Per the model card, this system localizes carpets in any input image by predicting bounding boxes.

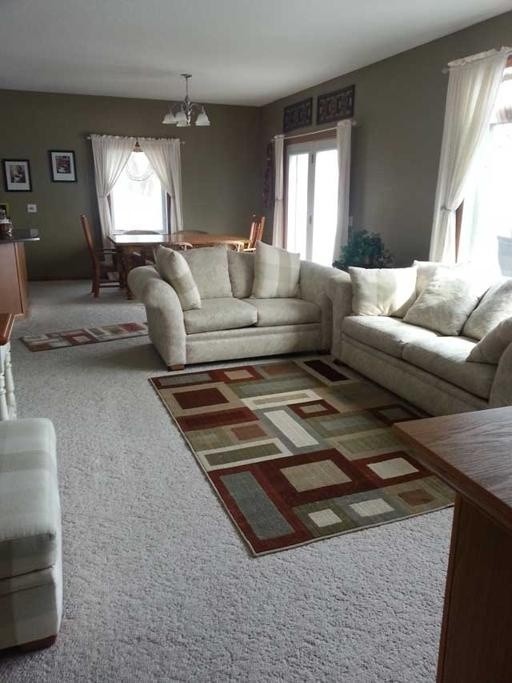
[148,351,458,557]
[19,319,149,352]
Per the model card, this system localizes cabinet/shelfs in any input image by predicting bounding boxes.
[391,405,512,682]
[1,240,30,318]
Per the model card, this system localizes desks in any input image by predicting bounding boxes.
[1,311,17,420]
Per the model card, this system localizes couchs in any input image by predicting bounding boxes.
[330,258,512,416]
[127,238,350,371]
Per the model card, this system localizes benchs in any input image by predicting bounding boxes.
[0,418,65,655]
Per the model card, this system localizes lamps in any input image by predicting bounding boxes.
[160,72,212,129]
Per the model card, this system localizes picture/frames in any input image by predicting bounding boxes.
[0,201,9,216]
[3,156,32,194]
[48,148,79,185]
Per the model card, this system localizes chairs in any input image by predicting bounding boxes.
[201,239,241,250]
[79,213,128,298]
[171,229,206,234]
[154,240,194,261]
[122,229,160,260]
[244,213,267,251]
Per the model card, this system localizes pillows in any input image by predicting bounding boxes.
[463,278,512,342]
[465,315,512,364]
[402,265,490,337]
[347,265,418,318]
[153,242,201,312]
[250,241,302,300]
